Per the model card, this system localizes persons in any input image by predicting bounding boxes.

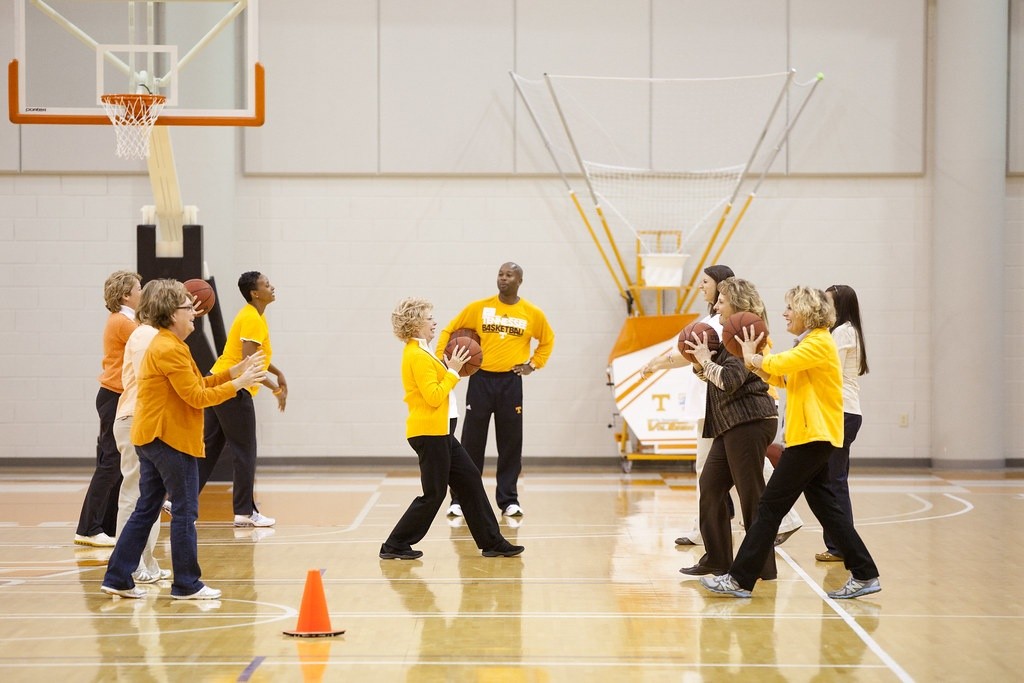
[675,265,804,547]
[99,281,267,600]
[815,285,869,561]
[378,298,525,560]
[163,271,288,527]
[697,286,881,604]
[74,271,143,547]
[113,278,204,583]
[678,277,777,581]
[434,262,556,514]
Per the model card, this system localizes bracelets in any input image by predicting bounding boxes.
[273,388,283,395]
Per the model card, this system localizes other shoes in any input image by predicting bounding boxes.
[674,537,695,545]
[814,551,844,562]
[773,526,803,547]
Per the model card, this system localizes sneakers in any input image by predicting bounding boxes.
[170,585,222,600]
[446,504,463,516]
[700,573,752,598]
[827,575,882,600]
[131,568,160,584]
[481,538,525,557]
[100,584,149,598]
[502,503,525,516]
[379,542,423,559]
[152,567,172,579]
[162,500,197,525]
[73,532,116,547]
[233,508,276,527]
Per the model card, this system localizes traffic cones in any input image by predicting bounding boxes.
[280,568,345,641]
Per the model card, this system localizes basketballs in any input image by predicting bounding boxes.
[678,322,720,363]
[722,311,768,357]
[443,337,483,377]
[448,327,481,346]
[182,279,216,319]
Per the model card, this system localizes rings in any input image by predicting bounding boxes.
[254,363,257,367]
[521,368,523,371]
[255,378,257,382]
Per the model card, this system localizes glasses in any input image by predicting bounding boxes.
[174,304,194,312]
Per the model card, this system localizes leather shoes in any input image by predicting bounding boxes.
[679,563,728,576]
